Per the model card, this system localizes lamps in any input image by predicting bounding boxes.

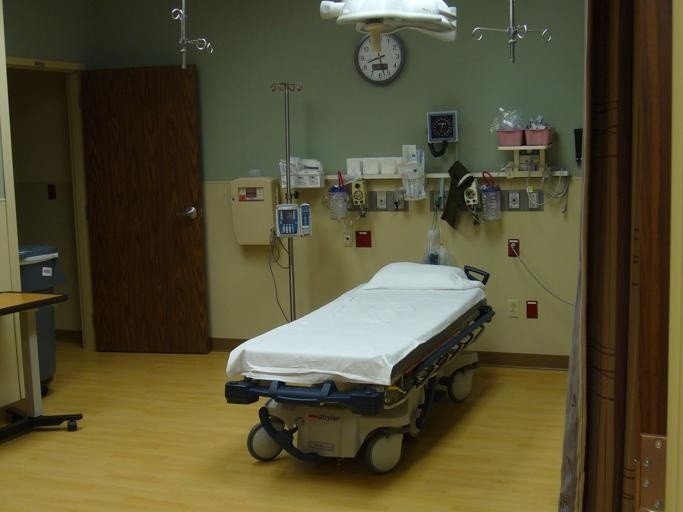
[319,0,457,42]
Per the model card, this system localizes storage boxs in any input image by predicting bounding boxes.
[496,130,525,146]
[525,128,553,145]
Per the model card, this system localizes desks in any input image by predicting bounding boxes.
[0,290,82,444]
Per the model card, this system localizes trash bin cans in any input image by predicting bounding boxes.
[17,244,59,396]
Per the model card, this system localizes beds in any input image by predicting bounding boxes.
[225,265,496,475]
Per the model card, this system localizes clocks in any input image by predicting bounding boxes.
[427,110,458,143]
[353,31,406,84]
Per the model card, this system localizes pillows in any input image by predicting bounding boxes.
[363,261,487,290]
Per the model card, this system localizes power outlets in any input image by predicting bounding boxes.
[507,239,519,257]
[433,195,443,208]
